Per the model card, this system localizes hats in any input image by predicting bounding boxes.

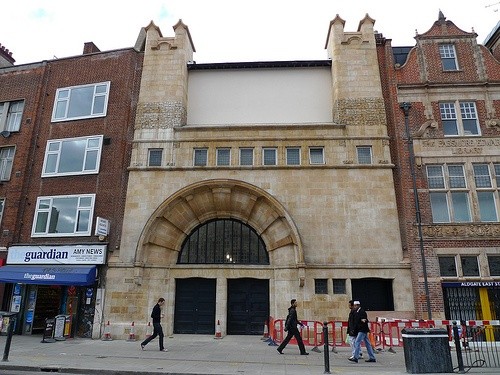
[354,301,360,305]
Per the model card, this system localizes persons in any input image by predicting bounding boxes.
[277,300,309,354]
[141,298,168,352]
[348,300,363,359]
[348,301,377,363]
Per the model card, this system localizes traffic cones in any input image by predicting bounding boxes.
[144,321,153,339]
[261,319,269,340]
[101,321,113,341]
[126,322,138,342]
[214,319,223,340]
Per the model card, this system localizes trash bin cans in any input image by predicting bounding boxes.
[0,311,17,336]
[401,327,454,373]
[53,314,72,341]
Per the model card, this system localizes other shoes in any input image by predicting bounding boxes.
[277,348,284,354]
[350,354,364,358]
[300,352,309,355]
[141,343,146,350]
[160,348,168,352]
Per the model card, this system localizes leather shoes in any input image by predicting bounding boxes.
[349,357,358,362]
[366,358,376,362]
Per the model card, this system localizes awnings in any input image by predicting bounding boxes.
[0,265,96,287]
